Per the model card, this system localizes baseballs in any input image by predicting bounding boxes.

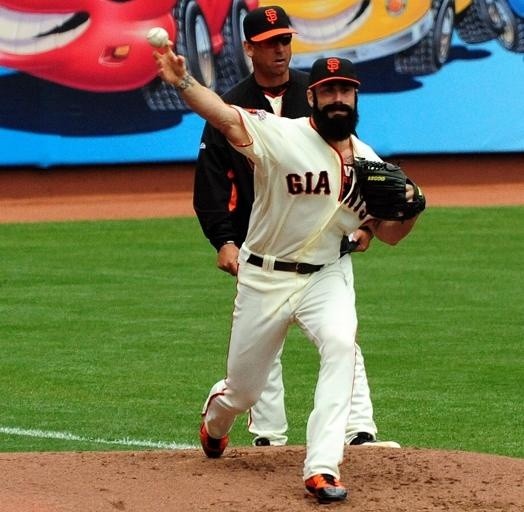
[148,27,168,49]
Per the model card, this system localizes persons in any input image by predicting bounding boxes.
[192,5,403,449]
[151,44,426,502]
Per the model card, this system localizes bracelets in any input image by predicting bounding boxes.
[223,240,235,244]
[176,73,192,92]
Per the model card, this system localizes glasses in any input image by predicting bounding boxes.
[256,35,291,48]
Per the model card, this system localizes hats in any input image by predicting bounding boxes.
[243,6,298,43]
[307,57,360,90]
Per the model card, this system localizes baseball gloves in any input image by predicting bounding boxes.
[351,158,425,222]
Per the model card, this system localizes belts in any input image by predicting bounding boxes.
[247,235,350,273]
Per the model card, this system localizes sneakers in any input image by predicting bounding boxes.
[304,474,347,498]
[348,432,401,448]
[200,420,229,459]
